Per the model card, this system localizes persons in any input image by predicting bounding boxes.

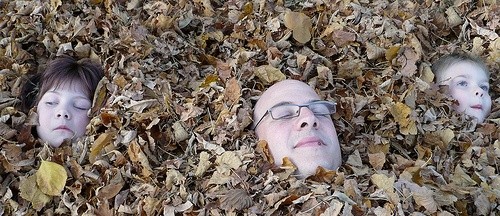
[33,53,105,149]
[251,79,342,182]
[430,54,493,126]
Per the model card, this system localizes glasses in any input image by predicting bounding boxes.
[252,101,335,136]
